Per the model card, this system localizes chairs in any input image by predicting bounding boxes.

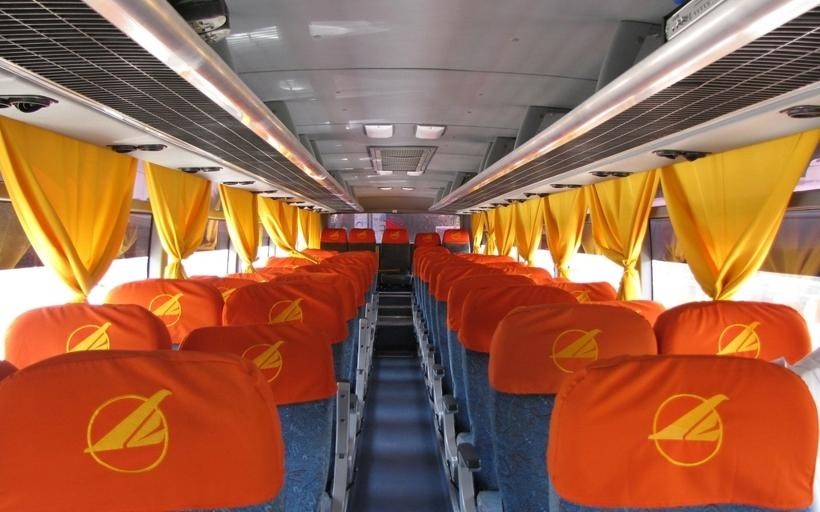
[379,229,412,288]
[2,247,380,511]
[414,231,441,245]
[409,245,819,512]
[319,228,348,252]
[346,229,377,250]
[443,229,472,253]
